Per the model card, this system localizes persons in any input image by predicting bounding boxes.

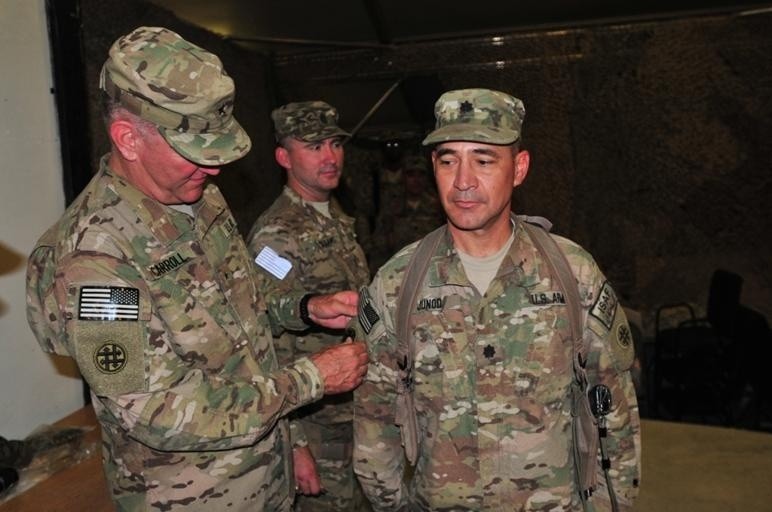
[349,87,642,511]
[25,27,369,511]
[372,155,446,277]
[245,101,376,511]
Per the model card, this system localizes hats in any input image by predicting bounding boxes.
[271,101,354,143]
[98,26,253,168]
[422,89,527,147]
